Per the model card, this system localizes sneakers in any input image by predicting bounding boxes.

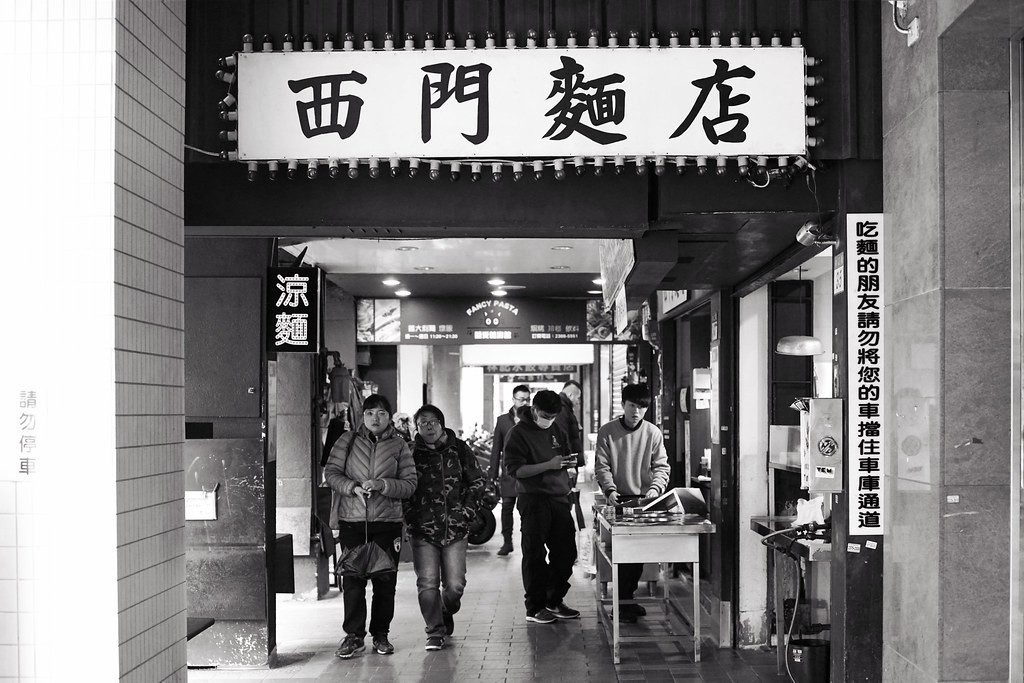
[334,632,365,658]
[525,606,557,624]
[372,634,394,655]
[544,601,580,618]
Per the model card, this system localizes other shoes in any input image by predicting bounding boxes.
[611,603,646,623]
[425,635,443,650]
[498,540,514,555]
[442,608,454,636]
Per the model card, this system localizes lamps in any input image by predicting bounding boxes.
[774,265,827,356]
[796,218,843,250]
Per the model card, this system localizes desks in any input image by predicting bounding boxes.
[592,504,716,665]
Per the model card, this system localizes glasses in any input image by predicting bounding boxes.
[363,409,388,418]
[417,418,441,428]
[514,396,531,402]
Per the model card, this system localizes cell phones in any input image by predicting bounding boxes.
[568,453,579,462]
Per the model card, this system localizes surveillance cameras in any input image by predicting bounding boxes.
[796,221,821,246]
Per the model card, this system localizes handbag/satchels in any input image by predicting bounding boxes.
[329,489,342,529]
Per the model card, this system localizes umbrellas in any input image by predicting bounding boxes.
[333,494,399,575]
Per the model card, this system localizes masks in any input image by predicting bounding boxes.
[533,409,556,430]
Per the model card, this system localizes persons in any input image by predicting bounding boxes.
[595,384,671,624]
[555,380,585,466]
[325,394,417,658]
[488,385,530,557]
[400,404,486,650]
[503,390,579,623]
